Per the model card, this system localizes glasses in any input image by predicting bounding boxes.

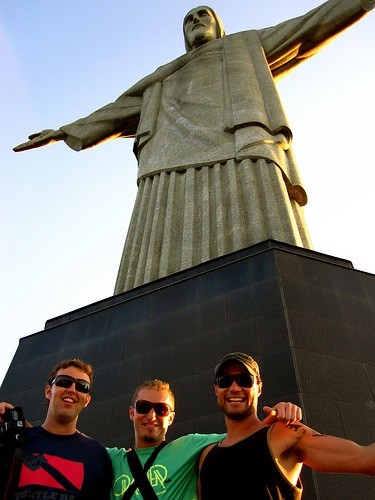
[51,375,92,393]
[215,374,259,388]
[134,399,173,416]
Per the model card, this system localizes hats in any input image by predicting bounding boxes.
[214,352,260,380]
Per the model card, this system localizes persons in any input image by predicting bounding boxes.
[195,352,375,500]
[0,377,302,500]
[11,0,375,296]
[0,357,115,500]
